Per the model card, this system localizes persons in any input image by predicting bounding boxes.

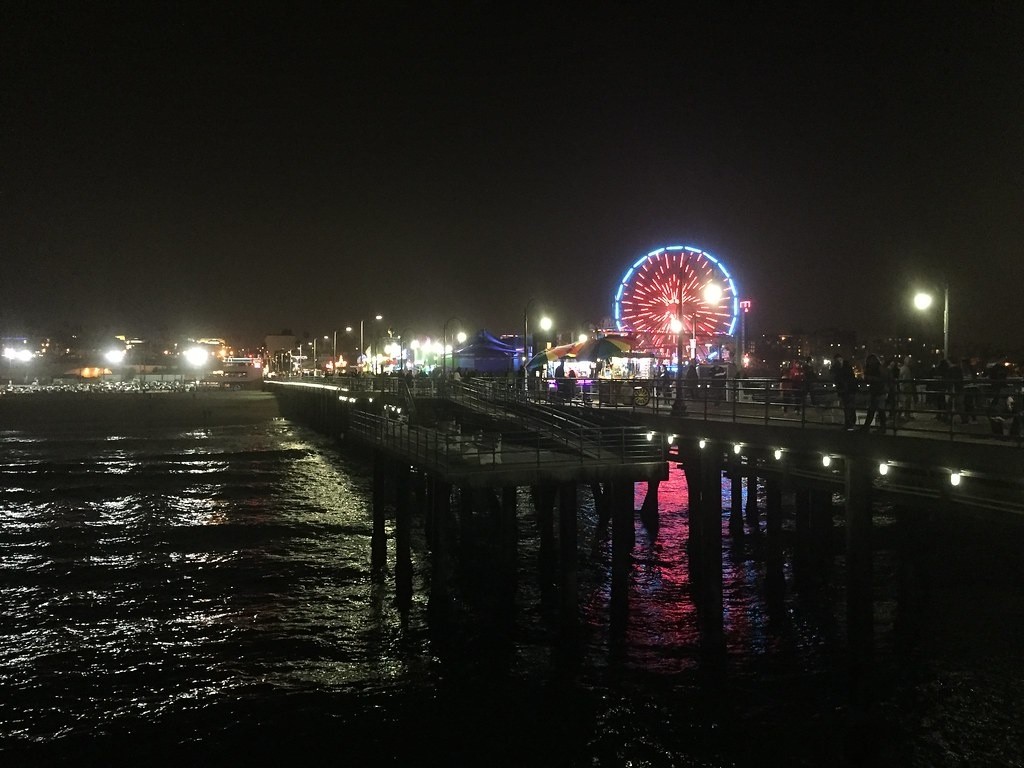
[311,345,1024,441]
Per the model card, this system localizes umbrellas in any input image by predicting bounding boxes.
[523,333,641,380]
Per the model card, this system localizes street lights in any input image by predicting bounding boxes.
[401,328,420,372]
[314,335,328,371]
[443,316,468,385]
[333,325,352,376]
[290,341,312,377]
[913,280,948,364]
[361,315,383,378]
[524,297,552,369]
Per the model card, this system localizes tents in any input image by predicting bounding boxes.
[446,328,520,375]
